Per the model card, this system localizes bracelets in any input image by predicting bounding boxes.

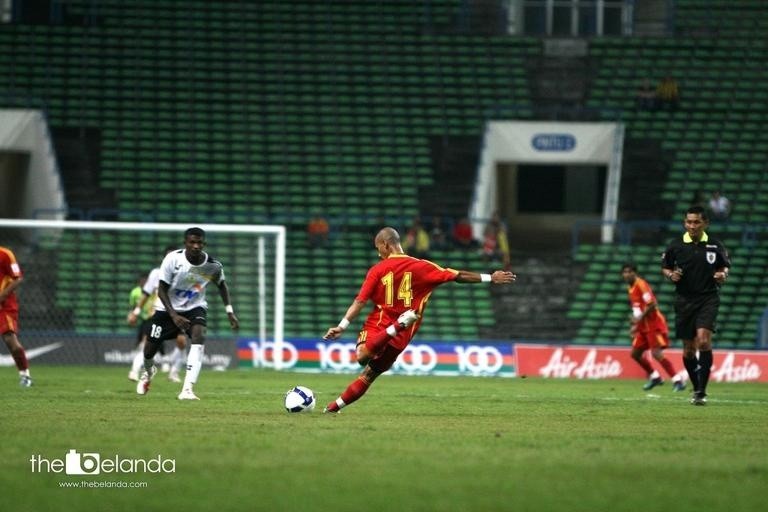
[667,271,672,279]
[338,318,350,330]
[480,273,491,282]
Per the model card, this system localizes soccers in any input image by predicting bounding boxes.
[284,386,315,413]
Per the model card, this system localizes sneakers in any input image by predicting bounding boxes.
[323,404,342,415]
[671,376,688,395]
[136,366,159,395]
[127,368,140,382]
[643,376,665,391]
[397,307,419,333]
[176,388,201,403]
[690,392,708,407]
[168,372,182,385]
[19,373,33,391]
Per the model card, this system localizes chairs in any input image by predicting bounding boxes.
[0,0,767,351]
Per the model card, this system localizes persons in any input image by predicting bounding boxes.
[660,207,732,405]
[621,263,687,392]
[323,227,516,413]
[365,211,503,263]
[705,190,734,224]
[128,227,239,400]
[0,247,32,387]
